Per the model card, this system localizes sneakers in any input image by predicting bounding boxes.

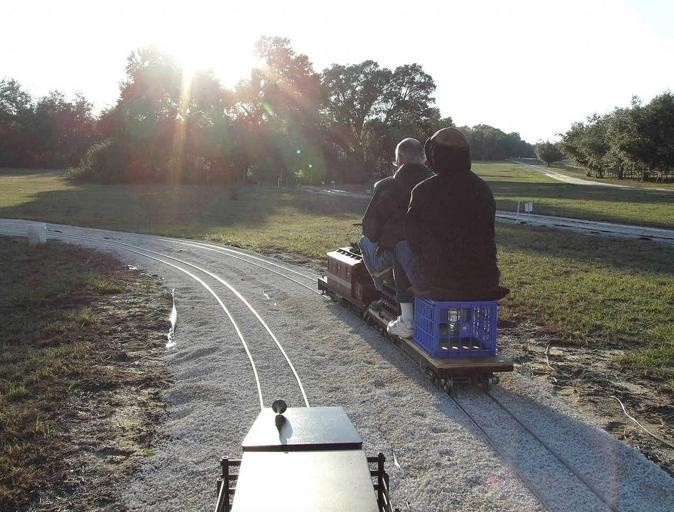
[387,315,416,338]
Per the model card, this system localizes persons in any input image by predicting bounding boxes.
[356,126,511,339]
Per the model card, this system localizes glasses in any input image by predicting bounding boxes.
[393,158,399,167]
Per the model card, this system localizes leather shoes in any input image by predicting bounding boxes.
[371,298,384,311]
